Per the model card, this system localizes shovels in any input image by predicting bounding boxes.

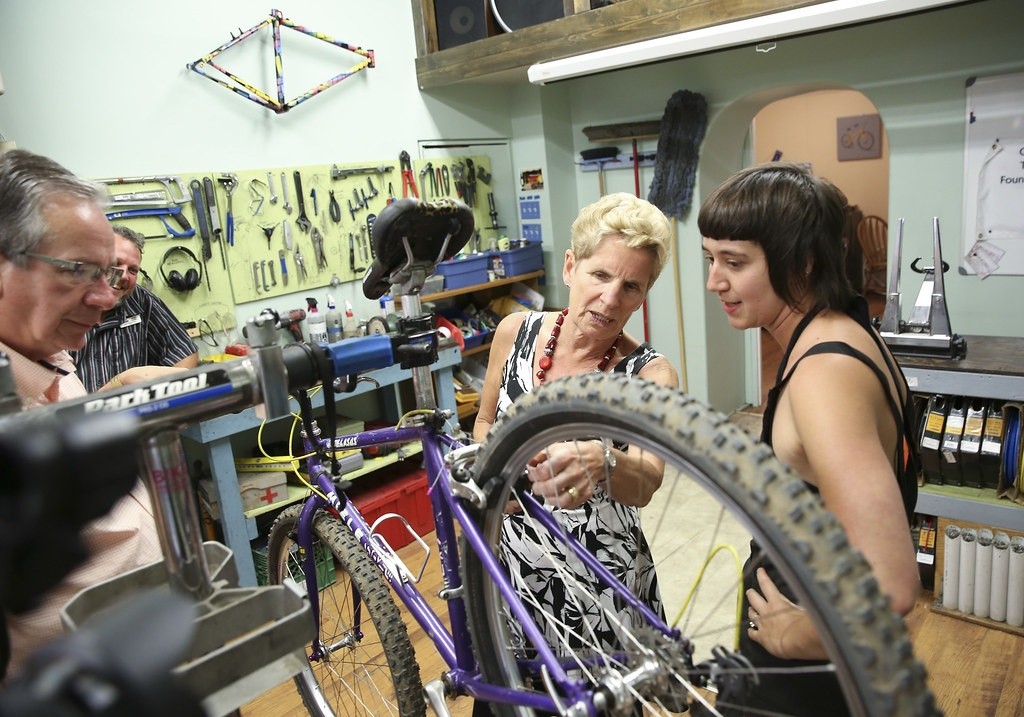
[327,190,342,222]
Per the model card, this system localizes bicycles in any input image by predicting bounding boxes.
[1,197,941,717]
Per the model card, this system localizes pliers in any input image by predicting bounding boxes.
[399,149,419,200]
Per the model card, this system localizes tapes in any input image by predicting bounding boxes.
[437,326,451,339]
[449,318,480,339]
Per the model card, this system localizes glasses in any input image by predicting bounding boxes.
[21,252,123,288]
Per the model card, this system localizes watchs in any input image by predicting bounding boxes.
[587,439,617,486]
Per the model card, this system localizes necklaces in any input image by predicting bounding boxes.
[536,308,622,387]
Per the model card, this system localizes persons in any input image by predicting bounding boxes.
[698,165,918,716]
[0,149,199,676]
[471,194,680,717]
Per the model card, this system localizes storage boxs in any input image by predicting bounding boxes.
[432,252,489,290]
[252,537,336,600]
[338,465,438,551]
[485,240,544,277]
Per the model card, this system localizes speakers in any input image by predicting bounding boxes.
[433,0,503,52]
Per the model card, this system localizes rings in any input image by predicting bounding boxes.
[566,486,580,508]
[749,614,757,627]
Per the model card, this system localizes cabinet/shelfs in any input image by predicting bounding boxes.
[389,270,546,422]
[176,343,462,590]
[868,336,1024,633]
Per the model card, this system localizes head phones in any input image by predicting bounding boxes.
[160,246,202,292]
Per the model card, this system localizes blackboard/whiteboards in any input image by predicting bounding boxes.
[958,71,1024,279]
